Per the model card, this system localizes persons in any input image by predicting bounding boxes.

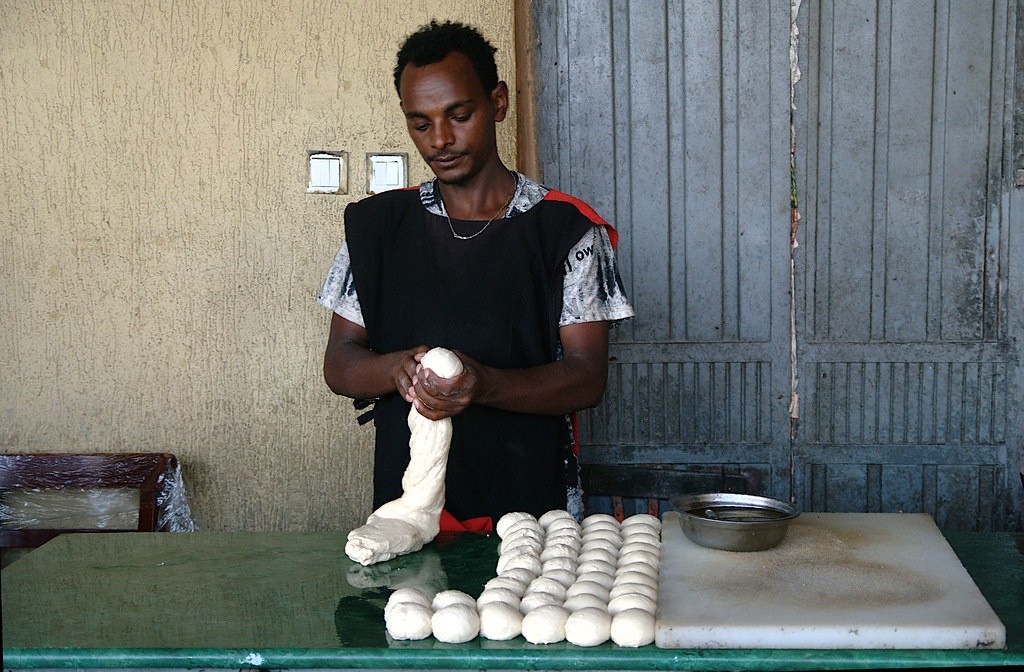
[317,19,635,534]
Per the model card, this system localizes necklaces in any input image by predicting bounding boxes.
[448,173,516,239]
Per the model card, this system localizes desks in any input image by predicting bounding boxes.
[2,531,1024,669]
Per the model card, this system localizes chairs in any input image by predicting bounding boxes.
[0,451,179,569]
[578,462,749,523]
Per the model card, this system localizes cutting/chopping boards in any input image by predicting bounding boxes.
[655,510,1005,650]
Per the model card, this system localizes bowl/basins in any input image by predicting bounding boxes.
[667,491,801,552]
